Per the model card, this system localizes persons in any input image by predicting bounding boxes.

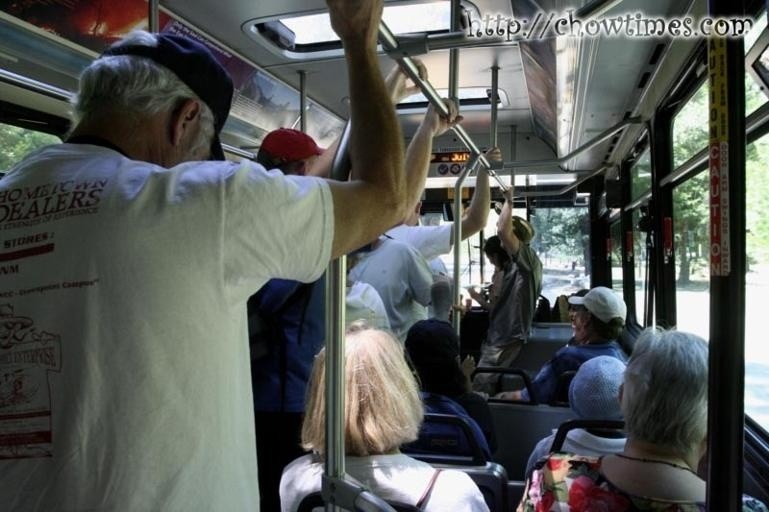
[341,97,630,406]
[513,324,766,510]
[2,2,408,511]
[397,319,495,448]
[245,56,470,509]
[524,355,629,481]
[279,320,491,512]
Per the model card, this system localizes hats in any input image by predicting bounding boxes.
[567,285,629,327]
[261,128,323,159]
[98,32,235,163]
[511,215,535,242]
[570,354,627,420]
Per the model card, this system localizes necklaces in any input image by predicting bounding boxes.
[614,452,699,474]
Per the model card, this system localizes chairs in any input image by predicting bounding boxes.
[295,365,769,512]
